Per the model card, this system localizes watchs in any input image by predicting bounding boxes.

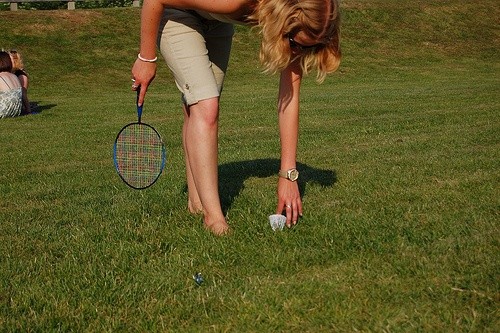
[278,168,299,182]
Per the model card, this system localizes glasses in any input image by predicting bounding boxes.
[289,32,321,50]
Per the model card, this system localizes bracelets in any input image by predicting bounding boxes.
[137,53,158,62]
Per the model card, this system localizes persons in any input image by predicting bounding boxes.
[0,50,30,118]
[130,0,342,238]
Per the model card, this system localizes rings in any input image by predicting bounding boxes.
[284,205,292,208]
[132,85,137,88]
[131,79,135,81]
